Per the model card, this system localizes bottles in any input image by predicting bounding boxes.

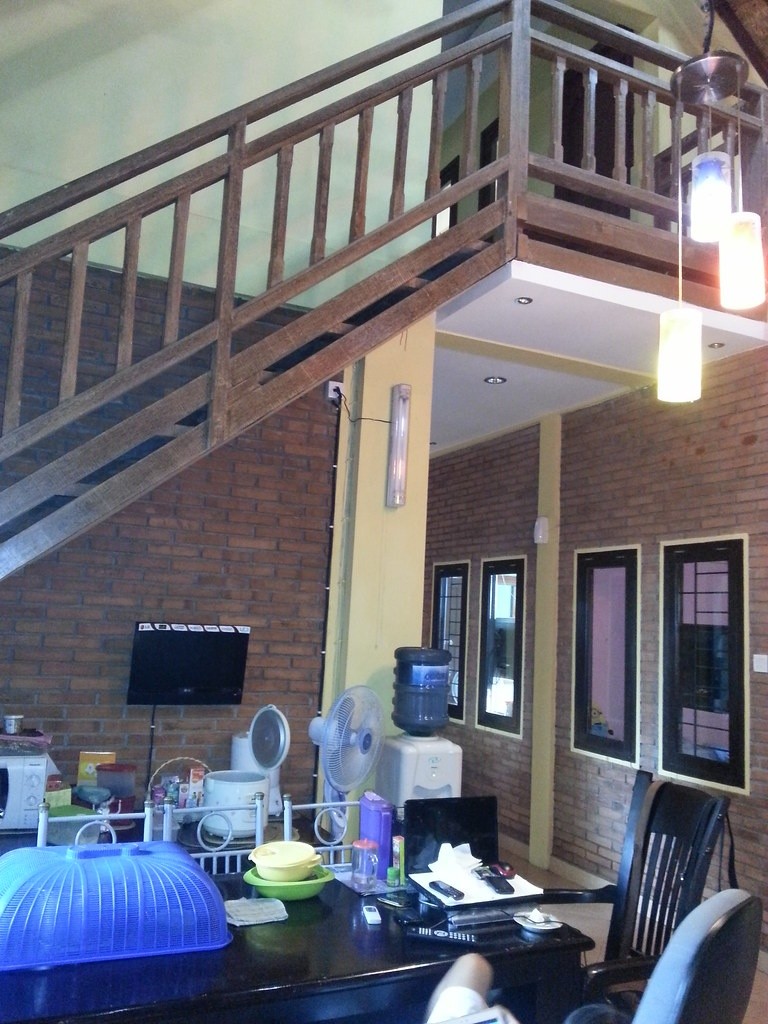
[392,647,452,736]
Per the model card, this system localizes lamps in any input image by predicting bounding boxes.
[386,383,412,508]
[656,0,766,403]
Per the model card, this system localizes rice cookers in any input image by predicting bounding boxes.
[203,704,291,840]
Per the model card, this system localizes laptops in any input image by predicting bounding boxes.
[402,795,545,909]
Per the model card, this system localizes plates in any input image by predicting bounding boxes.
[514,912,563,932]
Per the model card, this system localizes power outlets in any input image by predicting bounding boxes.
[325,380,343,401]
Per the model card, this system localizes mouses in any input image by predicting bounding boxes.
[489,862,516,879]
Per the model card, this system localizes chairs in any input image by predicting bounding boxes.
[564,888,763,1024]
[163,791,265,874]
[534,769,730,1024]
[283,789,374,864]
[37,801,155,847]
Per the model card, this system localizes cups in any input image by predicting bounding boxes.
[353,840,379,893]
[3,714,23,736]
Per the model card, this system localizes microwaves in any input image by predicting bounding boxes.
[0,747,47,829]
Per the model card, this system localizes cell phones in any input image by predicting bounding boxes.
[394,907,423,926]
[429,881,466,901]
[486,876,515,894]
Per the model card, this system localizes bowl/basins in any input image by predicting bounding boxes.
[243,865,335,901]
[48,806,101,846]
[248,841,321,881]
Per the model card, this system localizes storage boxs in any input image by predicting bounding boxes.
[45,780,72,807]
[182,765,205,788]
[46,805,103,846]
[178,782,190,808]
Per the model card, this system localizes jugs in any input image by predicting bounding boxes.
[359,791,392,880]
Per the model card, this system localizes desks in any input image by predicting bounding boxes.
[0,865,596,1024]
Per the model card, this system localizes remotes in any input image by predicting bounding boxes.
[363,906,382,925]
[406,926,479,946]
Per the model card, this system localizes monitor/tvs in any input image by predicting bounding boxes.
[127,622,250,706]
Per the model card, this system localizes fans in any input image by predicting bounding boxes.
[308,684,384,864]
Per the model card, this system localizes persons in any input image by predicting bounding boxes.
[425,954,631,1024]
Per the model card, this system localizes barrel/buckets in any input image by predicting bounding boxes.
[96,764,136,831]
